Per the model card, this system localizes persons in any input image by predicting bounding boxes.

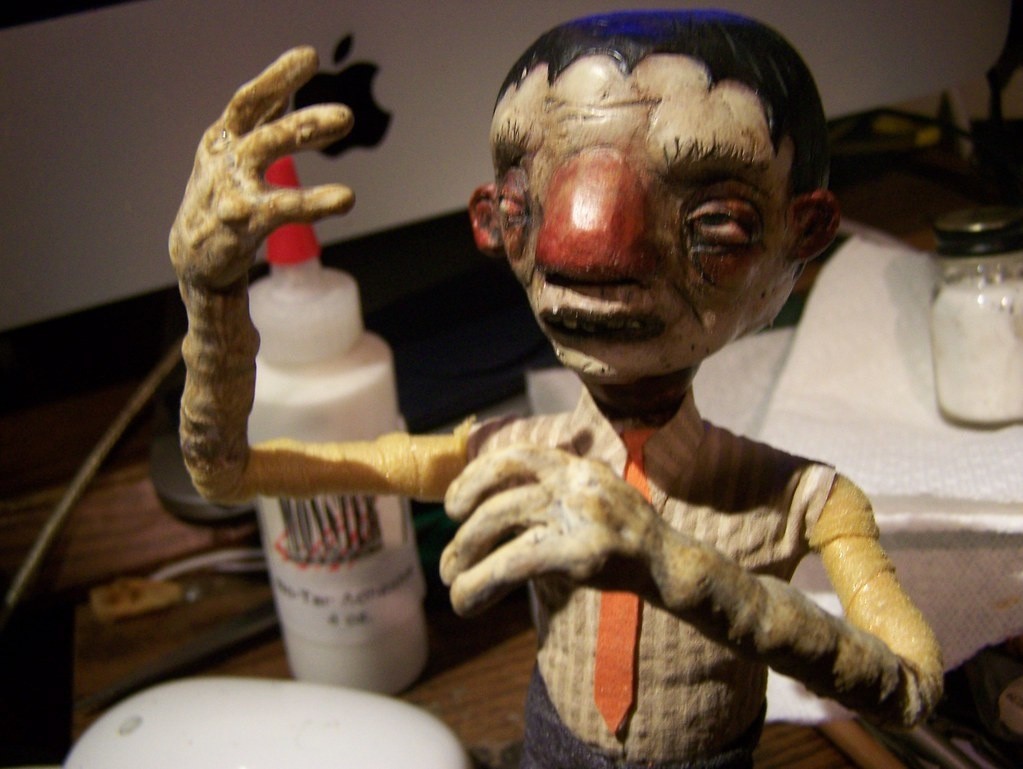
[166,9,945,769]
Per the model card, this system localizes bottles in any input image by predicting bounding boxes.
[929,208,1023,427]
[240,155,427,693]
